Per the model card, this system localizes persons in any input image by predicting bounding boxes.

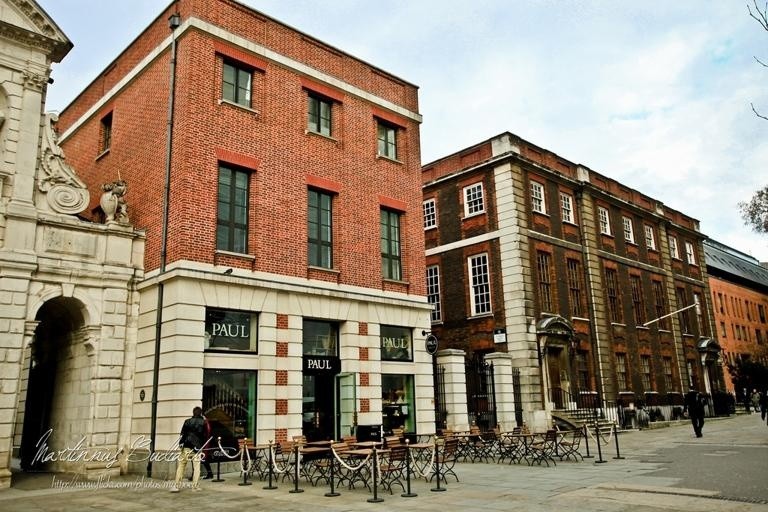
[170,407,206,493]
[741,388,751,412]
[202,415,213,479]
[759,391,768,420]
[683,386,704,437]
[750,388,760,413]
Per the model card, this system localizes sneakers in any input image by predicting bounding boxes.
[191,488,202,492]
[171,487,179,492]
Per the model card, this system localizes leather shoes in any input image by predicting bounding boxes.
[203,474,213,479]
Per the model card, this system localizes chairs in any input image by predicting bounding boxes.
[237,424,585,495]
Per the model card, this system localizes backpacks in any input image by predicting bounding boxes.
[696,392,707,406]
